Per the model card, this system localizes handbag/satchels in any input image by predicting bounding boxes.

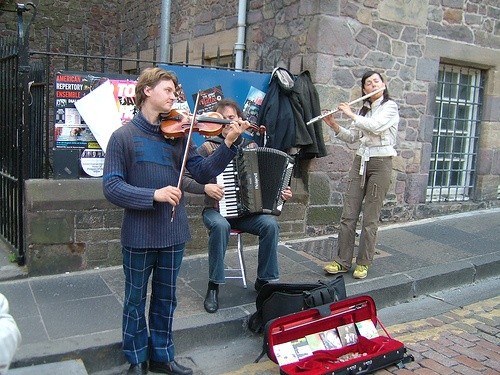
[247,275,347,335]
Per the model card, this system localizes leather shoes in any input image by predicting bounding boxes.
[149,359,193,375]
[203,289,220,313]
[254,278,269,291]
[126,360,148,375]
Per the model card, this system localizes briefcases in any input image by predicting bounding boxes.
[256,294,407,375]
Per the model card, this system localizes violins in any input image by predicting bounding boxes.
[158,108,267,140]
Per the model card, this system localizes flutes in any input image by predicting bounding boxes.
[308,87,386,126]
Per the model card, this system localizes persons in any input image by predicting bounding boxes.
[182,99,292,313]
[103,67,250,375]
[321,71,399,279]
[0,292,21,375]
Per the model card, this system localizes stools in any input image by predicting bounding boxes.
[208,228,247,289]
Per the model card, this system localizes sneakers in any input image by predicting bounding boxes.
[352,265,368,279]
[324,260,348,274]
